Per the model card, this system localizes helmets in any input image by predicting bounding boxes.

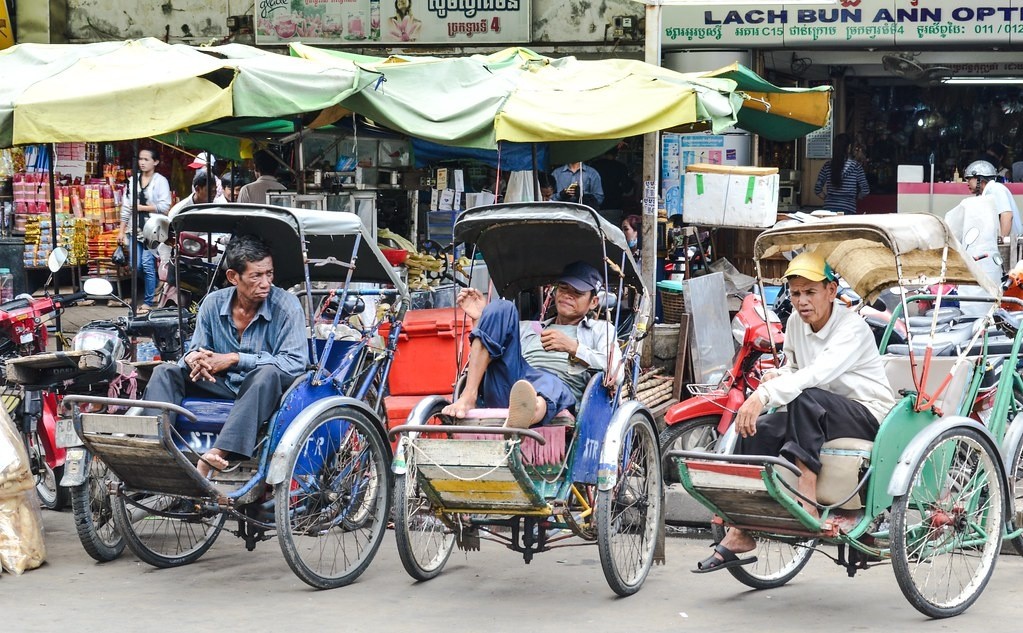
[964,160,998,176]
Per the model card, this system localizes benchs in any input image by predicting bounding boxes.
[454,370,614,462]
[773,354,967,513]
[174,342,363,426]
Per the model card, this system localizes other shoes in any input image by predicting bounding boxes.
[504,380,537,445]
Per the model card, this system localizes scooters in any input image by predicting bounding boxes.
[653,285,790,488]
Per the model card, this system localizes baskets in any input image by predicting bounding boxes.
[659,289,686,324]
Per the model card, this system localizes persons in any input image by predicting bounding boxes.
[117,146,290,314]
[386,0,422,43]
[551,161,604,208]
[814,133,871,217]
[143,236,309,481]
[534,173,557,201]
[441,260,625,443]
[621,215,643,284]
[695,253,898,573]
[964,160,1023,238]
[479,163,509,204]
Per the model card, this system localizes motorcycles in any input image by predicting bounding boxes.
[0,245,206,561]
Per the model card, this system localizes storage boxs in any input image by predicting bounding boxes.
[382,396,453,454]
[683,158,780,228]
[382,308,479,391]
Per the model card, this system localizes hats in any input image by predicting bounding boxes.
[557,262,604,295]
[187,152,216,169]
[780,252,834,282]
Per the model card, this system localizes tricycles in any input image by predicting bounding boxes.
[54,201,416,594]
[675,211,1023,619]
[379,204,665,597]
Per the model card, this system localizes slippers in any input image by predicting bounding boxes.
[690,544,758,574]
[137,307,151,315]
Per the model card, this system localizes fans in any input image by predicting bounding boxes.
[914,63,954,88]
[881,46,923,79]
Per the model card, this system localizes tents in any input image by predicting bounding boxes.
[0,37,835,156]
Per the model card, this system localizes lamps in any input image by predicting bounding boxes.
[941,76,1023,84]
